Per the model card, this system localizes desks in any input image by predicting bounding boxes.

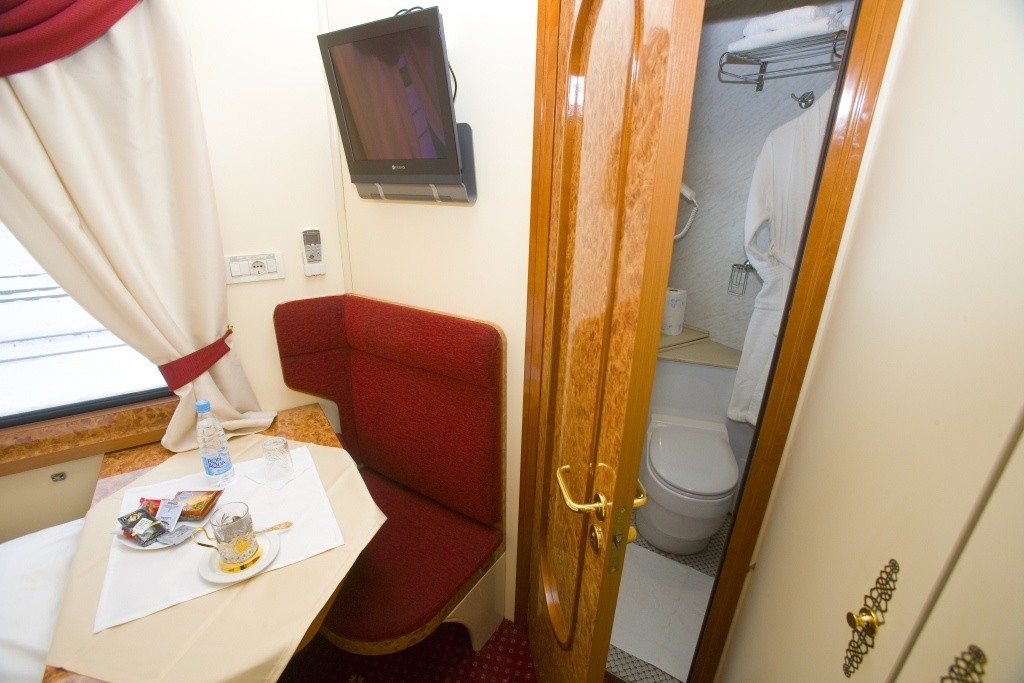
[654,320,742,367]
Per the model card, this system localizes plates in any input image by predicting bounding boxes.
[196,527,280,583]
[114,495,218,549]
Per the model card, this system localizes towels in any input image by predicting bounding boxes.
[742,6,834,37]
[727,15,844,63]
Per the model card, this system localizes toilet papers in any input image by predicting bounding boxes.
[660,287,688,336]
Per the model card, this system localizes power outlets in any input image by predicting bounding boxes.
[248,253,268,280]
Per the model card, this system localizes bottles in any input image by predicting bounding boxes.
[196,399,235,494]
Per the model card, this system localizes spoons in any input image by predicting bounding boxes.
[252,521,293,537]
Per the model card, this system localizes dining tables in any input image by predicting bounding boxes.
[43,404,384,683]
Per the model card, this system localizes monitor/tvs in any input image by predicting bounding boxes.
[317,6,464,185]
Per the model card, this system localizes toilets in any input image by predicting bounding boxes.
[638,413,741,556]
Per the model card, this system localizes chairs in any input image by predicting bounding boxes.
[274,291,508,657]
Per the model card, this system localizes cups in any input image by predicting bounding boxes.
[191,502,258,564]
[261,436,296,484]
